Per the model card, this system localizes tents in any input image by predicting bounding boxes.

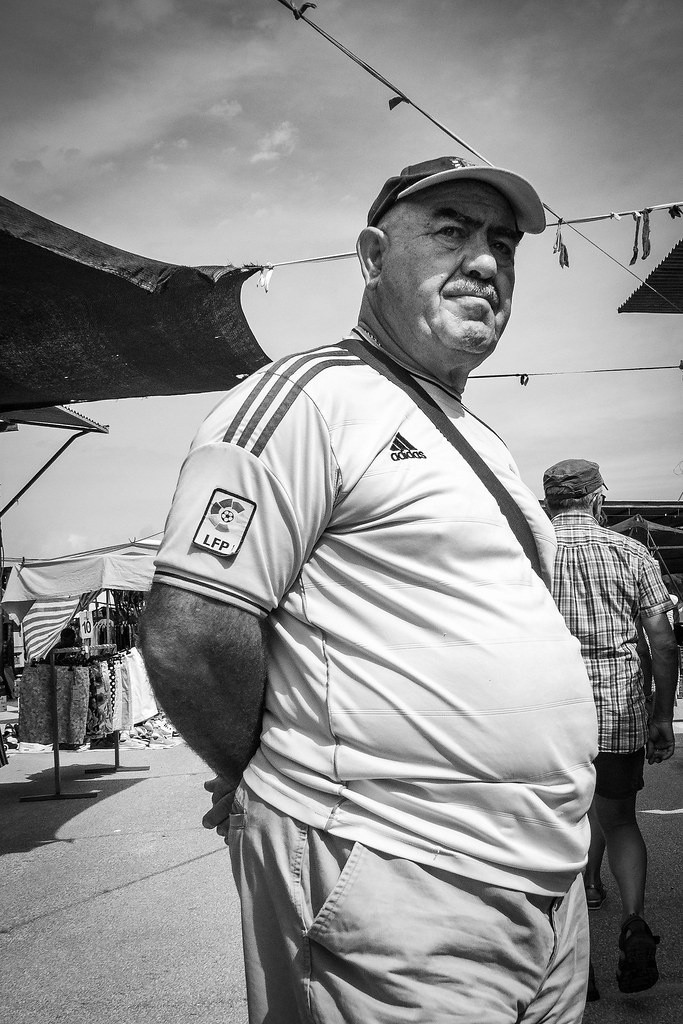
[1,538,165,655]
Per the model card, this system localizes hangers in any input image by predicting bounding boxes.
[30,645,131,668]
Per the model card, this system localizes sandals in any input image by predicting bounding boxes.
[587,959,600,1002]
[616,916,658,992]
[585,883,607,909]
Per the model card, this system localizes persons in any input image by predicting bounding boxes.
[44,628,79,666]
[141,154,604,1024]
[583,791,608,911]
[528,460,681,1001]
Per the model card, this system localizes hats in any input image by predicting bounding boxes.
[543,459,608,499]
[368,156,547,243]
